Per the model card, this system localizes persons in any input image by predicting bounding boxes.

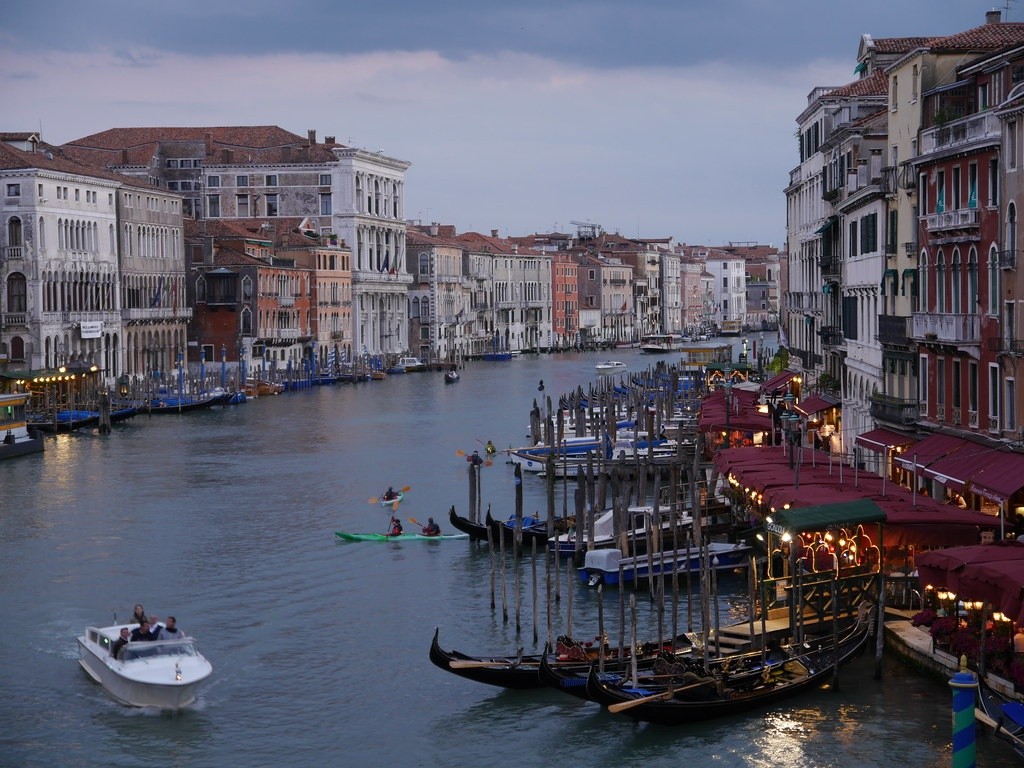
[380,515,402,536]
[466,451,483,465]
[886,475,967,511]
[383,487,398,501]
[1008,621,1024,652]
[764,384,788,413]
[484,441,496,453]
[418,518,441,536]
[113,604,184,660]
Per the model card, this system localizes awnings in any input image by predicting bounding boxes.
[761,370,797,392]
[793,396,833,419]
[854,428,1024,504]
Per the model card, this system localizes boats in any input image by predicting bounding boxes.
[76,613,213,708]
[586,603,877,725]
[466,453,494,471]
[381,491,404,507]
[537,599,869,703]
[508,371,725,479]
[449,504,613,549]
[428,602,776,691]
[0,358,428,433]
[595,360,627,375]
[547,505,693,567]
[489,351,522,357]
[577,543,754,595]
[334,531,469,542]
[640,334,683,353]
[444,364,460,383]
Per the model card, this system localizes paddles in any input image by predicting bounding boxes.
[408,516,426,528]
[628,674,686,678]
[388,500,399,532]
[455,449,494,467]
[608,677,720,714]
[622,685,682,687]
[475,438,503,455]
[522,517,563,529]
[449,660,590,668]
[369,485,411,504]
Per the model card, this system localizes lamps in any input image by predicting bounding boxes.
[938,588,1011,621]
[128,317,195,326]
